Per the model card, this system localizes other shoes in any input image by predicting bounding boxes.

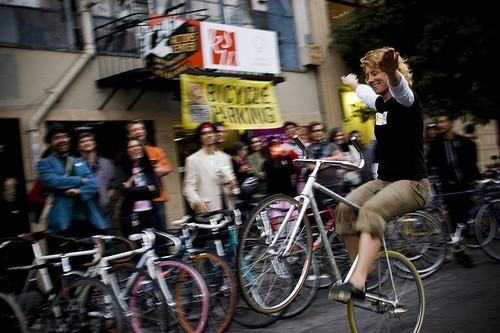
[333,282,365,301]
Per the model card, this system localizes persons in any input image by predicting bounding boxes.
[0,118,379,330]
[329,46,433,302]
[424,114,489,267]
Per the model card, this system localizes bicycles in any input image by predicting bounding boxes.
[111,190,394,333]
[26,231,210,333]
[0,229,124,333]
[235,152,425,333]
[377,175,500,281]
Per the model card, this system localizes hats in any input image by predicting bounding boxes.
[44,122,74,142]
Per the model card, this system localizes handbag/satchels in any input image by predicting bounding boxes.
[38,194,56,226]
